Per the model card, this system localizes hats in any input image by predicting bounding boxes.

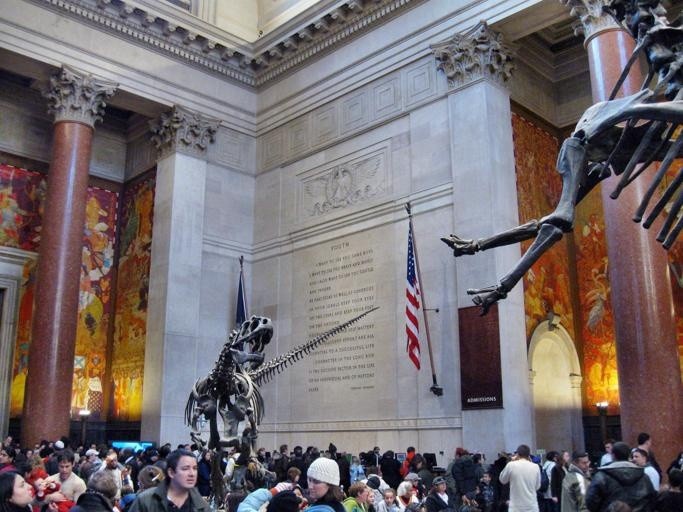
[404,473,422,480]
[305,456,342,486]
[86,449,100,457]
[55,441,65,449]
[432,476,446,485]
[149,450,160,457]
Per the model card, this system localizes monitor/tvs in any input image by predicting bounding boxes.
[397,453,406,463]
[423,452,436,467]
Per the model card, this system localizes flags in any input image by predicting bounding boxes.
[403,215,423,370]
[235,271,250,372]
